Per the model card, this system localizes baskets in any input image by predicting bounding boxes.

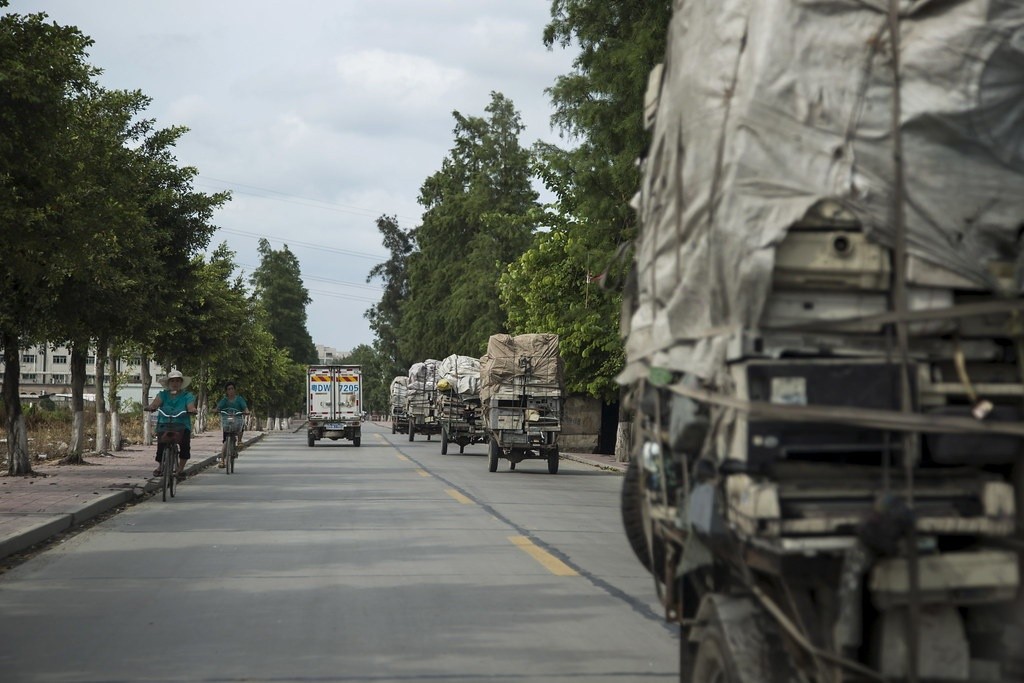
[221,416,245,435]
[156,422,184,444]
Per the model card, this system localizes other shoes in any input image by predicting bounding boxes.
[237,442,244,449]
[218,462,224,468]
[179,471,187,476]
[153,470,163,476]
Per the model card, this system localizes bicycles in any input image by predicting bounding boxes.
[210,408,254,474]
[144,407,197,502]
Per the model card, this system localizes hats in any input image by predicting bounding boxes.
[158,370,192,390]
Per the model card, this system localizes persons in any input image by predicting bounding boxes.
[144,370,198,480]
[211,383,249,468]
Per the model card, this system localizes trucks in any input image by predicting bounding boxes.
[408,359,444,442]
[389,376,409,435]
[304,364,362,447]
[593,0,1024,683]
[480,333,561,473]
[436,353,487,454]
